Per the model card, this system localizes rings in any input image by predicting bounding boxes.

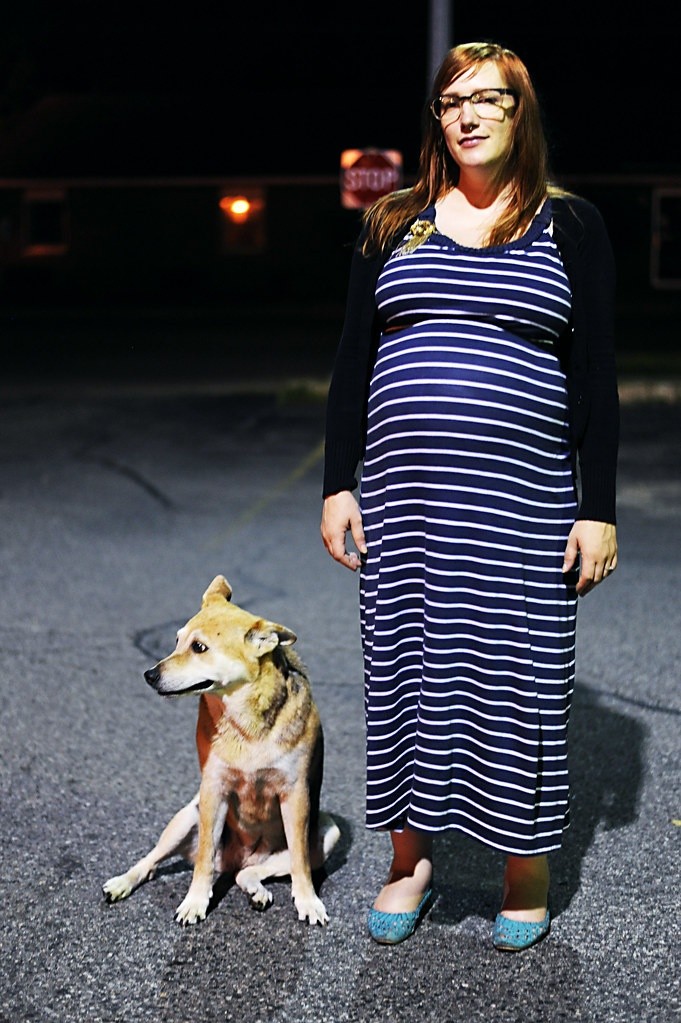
[605,567,613,570]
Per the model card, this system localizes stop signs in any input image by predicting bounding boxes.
[341,148,402,208]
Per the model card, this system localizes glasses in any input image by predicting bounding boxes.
[428,86,519,121]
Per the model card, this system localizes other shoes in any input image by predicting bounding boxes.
[491,901,551,951]
[365,881,437,946]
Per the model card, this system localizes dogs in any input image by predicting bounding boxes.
[100,574,342,927]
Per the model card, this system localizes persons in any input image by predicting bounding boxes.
[317,38,622,954]
